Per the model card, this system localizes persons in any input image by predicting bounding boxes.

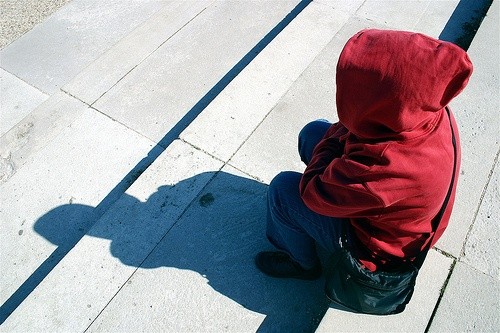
[256,29,472,279]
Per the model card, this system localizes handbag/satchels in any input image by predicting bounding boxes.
[325,238,420,315]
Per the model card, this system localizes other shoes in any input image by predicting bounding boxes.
[255,250,311,277]
